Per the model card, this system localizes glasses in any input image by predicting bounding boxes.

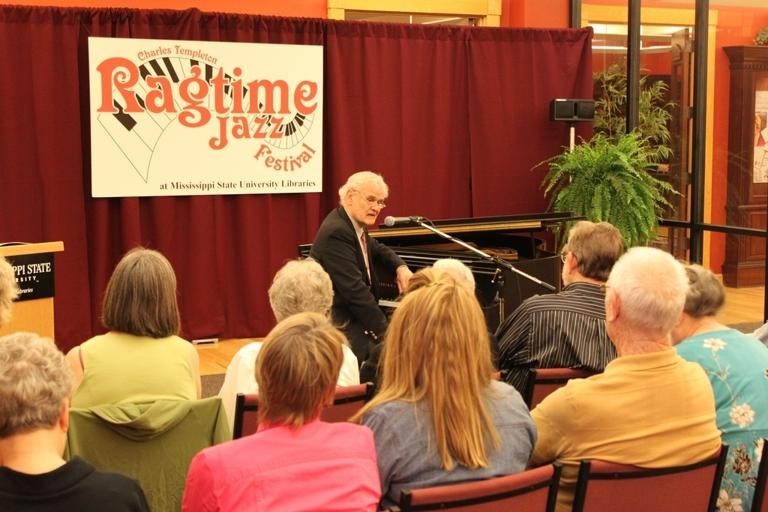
[355,190,387,210]
[561,249,580,264]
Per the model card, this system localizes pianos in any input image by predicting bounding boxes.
[298,214,570,332]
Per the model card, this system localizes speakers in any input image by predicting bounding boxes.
[552,98,596,122]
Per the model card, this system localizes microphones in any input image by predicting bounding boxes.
[384,215,423,227]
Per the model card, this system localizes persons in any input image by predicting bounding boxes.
[669,264,768,512]
[182,312,383,512]
[0,332,151,512]
[360,258,498,384]
[66,248,201,409]
[0,256,22,323]
[526,246,722,512]
[494,220,625,402]
[219,257,360,441]
[308,171,414,368]
[348,267,537,511]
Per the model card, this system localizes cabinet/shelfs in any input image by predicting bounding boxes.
[723,44,768,287]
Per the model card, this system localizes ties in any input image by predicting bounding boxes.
[360,232,371,287]
